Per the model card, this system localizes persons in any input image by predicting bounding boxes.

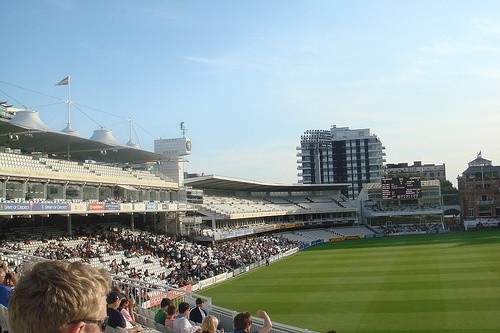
[8,259,111,333]
[201,315,218,333]
[234,310,272,333]
[0,196,500,333]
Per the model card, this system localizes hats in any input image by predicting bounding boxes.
[109,292,124,299]
[196,298,203,303]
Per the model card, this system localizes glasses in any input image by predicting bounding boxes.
[68,316,109,332]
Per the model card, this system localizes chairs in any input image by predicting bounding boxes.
[0,226,179,298]
[0,152,166,182]
[0,197,179,204]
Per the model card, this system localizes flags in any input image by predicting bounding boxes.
[478,152,480,155]
[57,76,68,85]
